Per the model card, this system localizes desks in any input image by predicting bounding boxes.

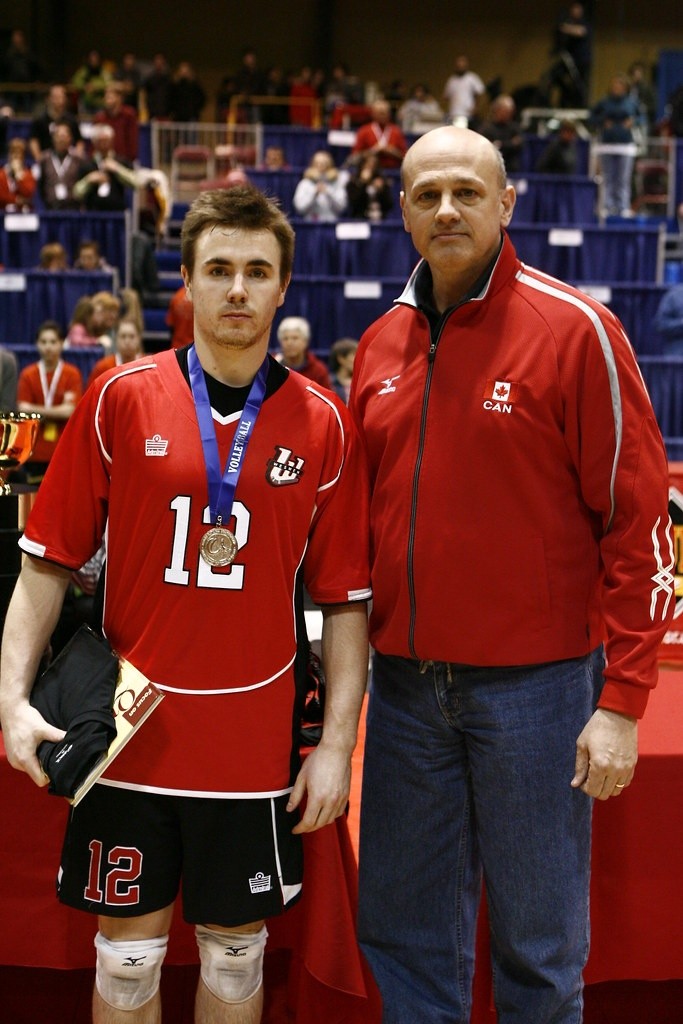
[0,732,383,1023]
[464,665,683,1024]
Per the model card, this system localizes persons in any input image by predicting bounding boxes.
[0,185,372,1024]
[0,29,208,306]
[167,286,195,350]
[331,339,358,405]
[0,288,151,461]
[270,317,331,391]
[218,53,524,221]
[536,1,683,221]
[348,128,677,1024]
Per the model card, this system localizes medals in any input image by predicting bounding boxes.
[200,528,238,567]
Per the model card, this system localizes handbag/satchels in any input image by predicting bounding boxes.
[28,622,118,798]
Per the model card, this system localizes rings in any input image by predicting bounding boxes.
[616,784,625,787]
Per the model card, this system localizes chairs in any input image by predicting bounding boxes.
[1,116,683,464]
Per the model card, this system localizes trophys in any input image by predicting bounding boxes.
[0,413,42,496]
